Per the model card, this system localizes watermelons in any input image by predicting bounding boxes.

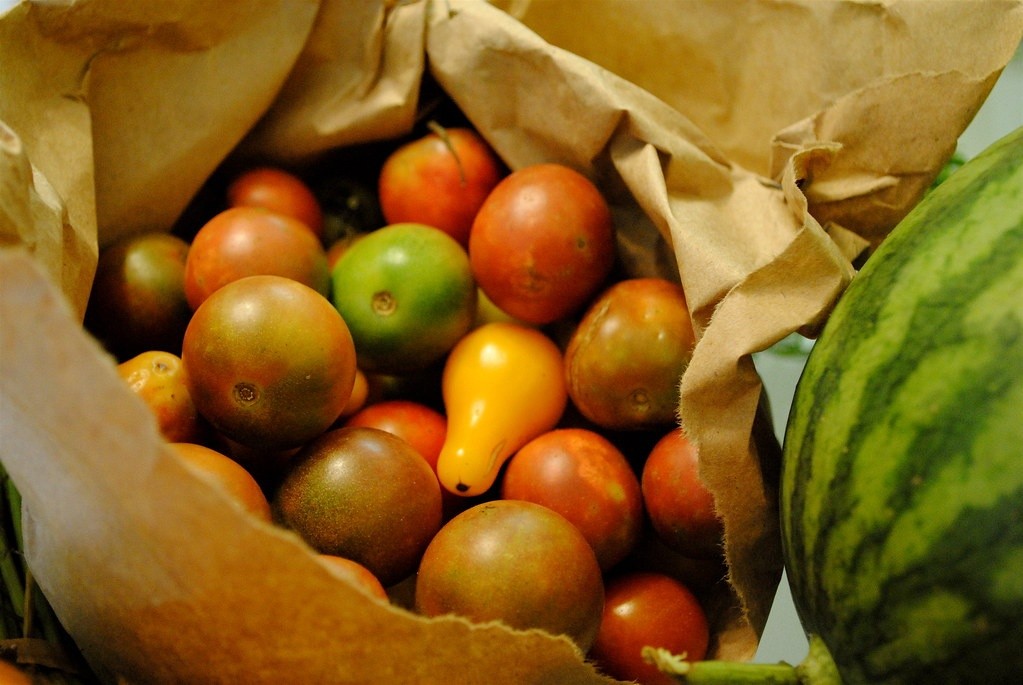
[640,125,1023,685]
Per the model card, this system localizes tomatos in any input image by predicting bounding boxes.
[90,127,727,683]
